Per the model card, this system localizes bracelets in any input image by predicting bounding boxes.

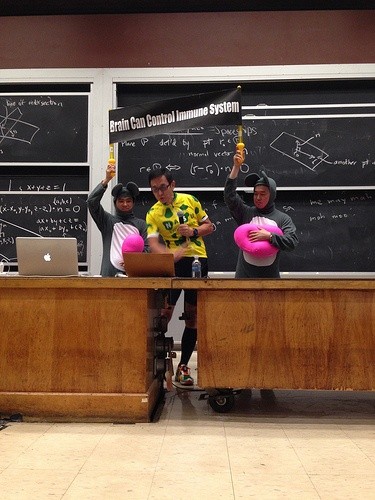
[270,232,272,242]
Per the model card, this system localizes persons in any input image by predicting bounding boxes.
[86,163,152,277]
[223,148,299,405]
[145,167,214,385]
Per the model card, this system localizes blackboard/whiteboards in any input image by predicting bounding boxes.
[116,77,375,278]
[0,81,90,274]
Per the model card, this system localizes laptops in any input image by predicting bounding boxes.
[123,251,175,277]
[15,237,88,276]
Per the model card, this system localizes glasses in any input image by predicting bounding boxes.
[151,183,171,191]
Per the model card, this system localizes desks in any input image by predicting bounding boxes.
[0,276,375,423]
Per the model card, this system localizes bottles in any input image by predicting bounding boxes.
[235,126,245,163]
[108,144,115,177]
[191,255,201,279]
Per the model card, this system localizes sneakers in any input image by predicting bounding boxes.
[175,366,194,385]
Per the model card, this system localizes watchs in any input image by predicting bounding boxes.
[191,227,198,237]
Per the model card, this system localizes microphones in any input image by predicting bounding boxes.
[177,211,186,241]
